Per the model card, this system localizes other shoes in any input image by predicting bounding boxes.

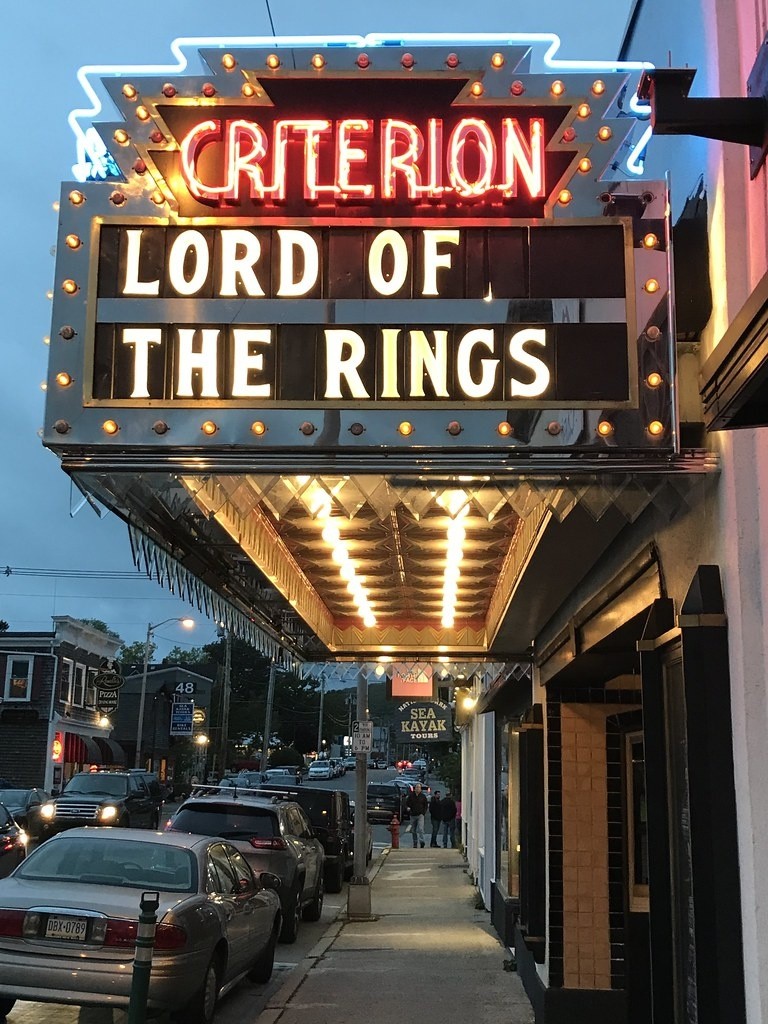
[444,845,447,848]
[421,843,424,847]
[431,843,440,848]
[452,844,458,849]
[413,844,417,848]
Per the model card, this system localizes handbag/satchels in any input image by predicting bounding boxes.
[406,824,412,832]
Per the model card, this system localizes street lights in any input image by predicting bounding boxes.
[134,618,194,769]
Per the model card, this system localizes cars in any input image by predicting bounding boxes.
[365,757,432,824]
[221,765,303,787]
[0,788,51,877]
[309,756,387,780]
[0,825,284,1024]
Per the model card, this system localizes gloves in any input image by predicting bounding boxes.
[407,807,411,811]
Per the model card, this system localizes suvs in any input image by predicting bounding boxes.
[163,781,374,944]
[40,765,164,836]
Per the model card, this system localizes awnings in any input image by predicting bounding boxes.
[92,736,124,763]
[65,732,103,765]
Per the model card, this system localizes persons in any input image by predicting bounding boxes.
[420,768,426,783]
[435,761,438,769]
[428,790,461,848]
[405,783,428,849]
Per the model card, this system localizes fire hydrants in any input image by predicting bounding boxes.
[386,815,401,849]
